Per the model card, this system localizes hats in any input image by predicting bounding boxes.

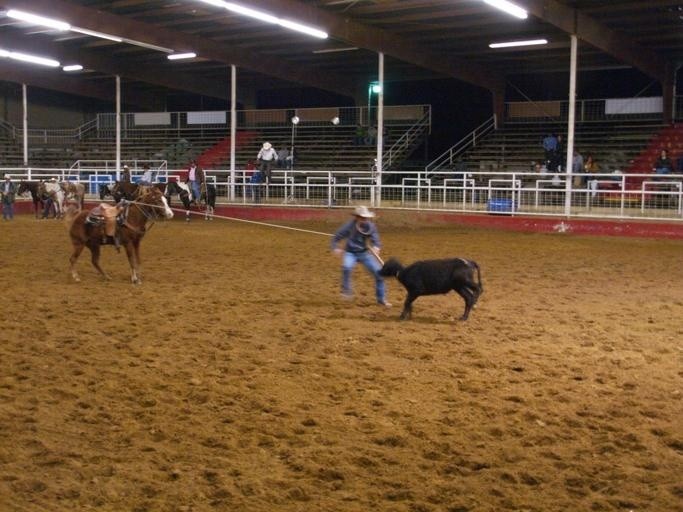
[351,206,375,218]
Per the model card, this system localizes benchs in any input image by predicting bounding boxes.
[0,123,424,181]
[434,112,683,204]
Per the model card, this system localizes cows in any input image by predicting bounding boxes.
[378,256,484,321]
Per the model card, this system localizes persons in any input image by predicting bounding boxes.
[257,142,278,180]
[42,178,55,219]
[185,158,204,202]
[277,143,289,168]
[332,205,393,306]
[0,174,16,223]
[123,165,130,183]
[530,131,597,188]
[354,122,377,144]
[653,148,673,173]
[136,165,153,185]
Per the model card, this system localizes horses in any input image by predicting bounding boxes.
[64,186,175,286]
[163,181,216,221]
[97,183,130,202]
[111,179,161,208]
[17,179,86,223]
